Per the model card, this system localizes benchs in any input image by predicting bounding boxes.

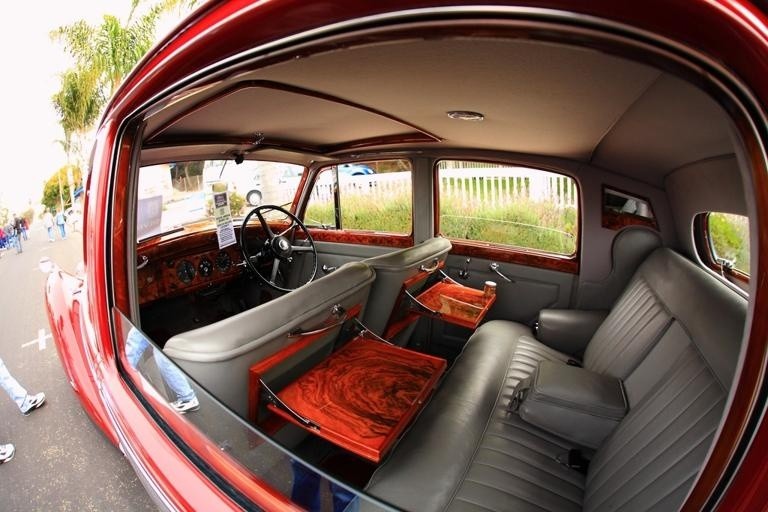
[161,232,452,477]
[355,245,751,511]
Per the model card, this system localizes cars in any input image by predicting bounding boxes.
[203,155,376,206]
[40,0,766,511]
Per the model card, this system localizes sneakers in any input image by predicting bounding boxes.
[20,392,46,416]
[169,397,200,415]
[0,443,15,464]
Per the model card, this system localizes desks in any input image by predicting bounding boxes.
[386,258,497,338]
[250,306,449,463]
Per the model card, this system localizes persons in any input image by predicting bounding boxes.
[1,359,45,465]
[42,207,68,242]
[125,327,200,415]
[0,214,28,254]
